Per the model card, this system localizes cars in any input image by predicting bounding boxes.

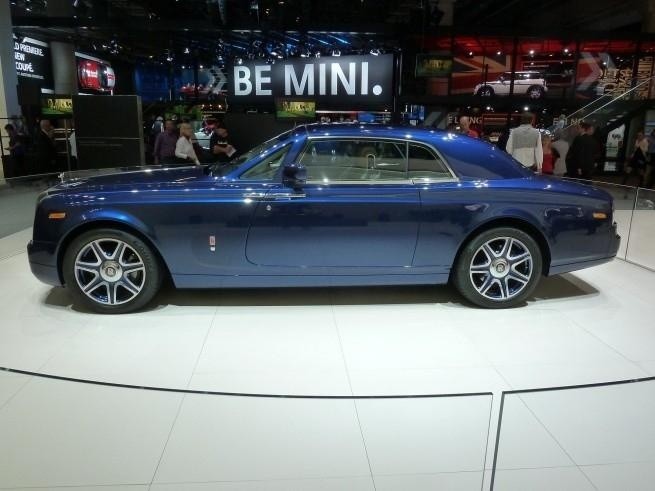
[473,70,550,102]
[26,121,622,313]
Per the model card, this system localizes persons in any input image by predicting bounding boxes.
[626,129,655,186]
[496,113,601,183]
[459,114,478,139]
[3,116,57,173]
[152,115,236,165]
[67,131,78,158]
